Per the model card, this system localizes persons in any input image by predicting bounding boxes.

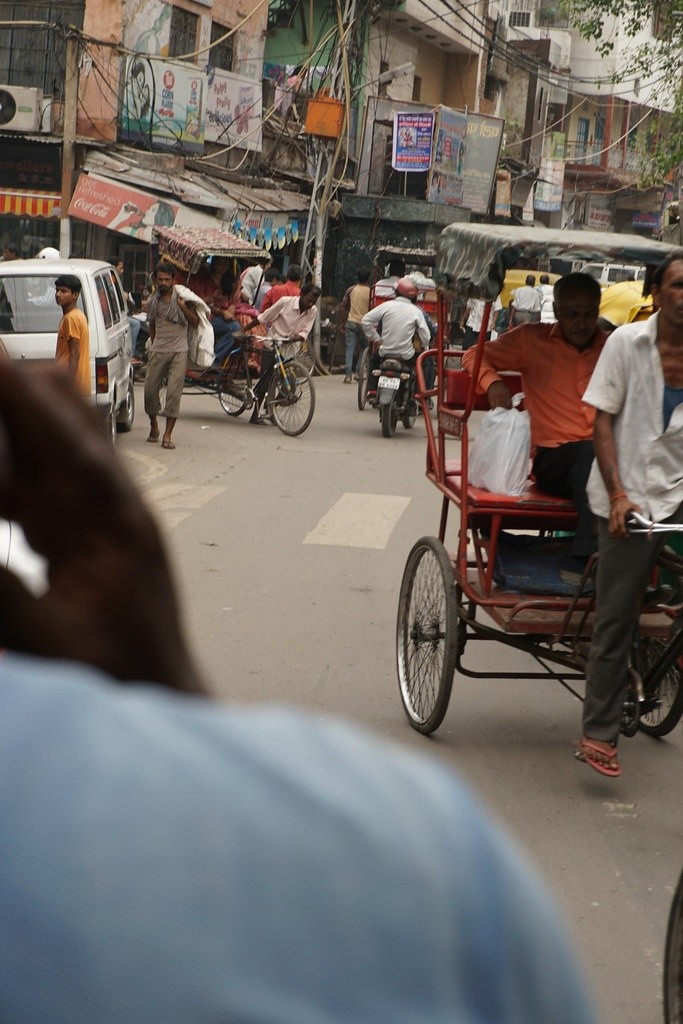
[0,353,591,1024]
[1,254,683,776]
[123,62,149,119]
[107,201,177,243]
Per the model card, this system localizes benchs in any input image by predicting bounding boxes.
[421,349,658,606]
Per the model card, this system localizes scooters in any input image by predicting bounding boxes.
[368,359,419,439]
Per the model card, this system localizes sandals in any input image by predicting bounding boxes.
[580,738,621,777]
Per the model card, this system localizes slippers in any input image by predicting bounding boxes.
[146,432,158,442]
[162,441,174,449]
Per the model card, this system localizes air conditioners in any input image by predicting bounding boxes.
[0,85,43,134]
[509,11,535,28]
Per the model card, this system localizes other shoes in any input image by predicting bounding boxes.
[367,393,376,400]
[343,377,351,383]
[353,372,364,382]
[248,419,266,425]
[130,357,143,366]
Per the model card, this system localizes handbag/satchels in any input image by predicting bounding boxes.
[467,393,530,495]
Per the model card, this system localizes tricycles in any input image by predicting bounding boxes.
[131,226,316,438]
[394,221,683,740]
[356,245,438,412]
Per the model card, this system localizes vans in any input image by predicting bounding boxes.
[579,262,646,294]
[0,257,135,456]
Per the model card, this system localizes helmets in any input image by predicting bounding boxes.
[38,247,61,260]
[394,278,419,298]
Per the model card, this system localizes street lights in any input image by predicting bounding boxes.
[311,61,416,373]
